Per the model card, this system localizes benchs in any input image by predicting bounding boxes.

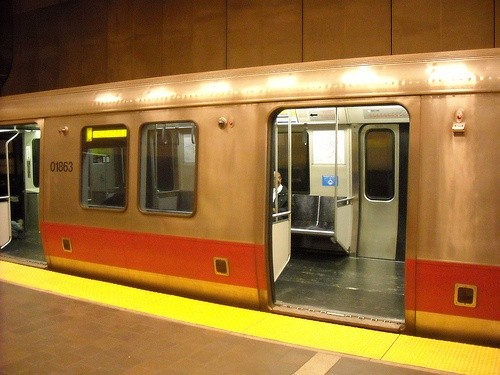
[290,194,351,256]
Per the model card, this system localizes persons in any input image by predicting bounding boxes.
[272,171,297,222]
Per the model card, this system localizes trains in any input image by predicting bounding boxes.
[0,47,500,347]
